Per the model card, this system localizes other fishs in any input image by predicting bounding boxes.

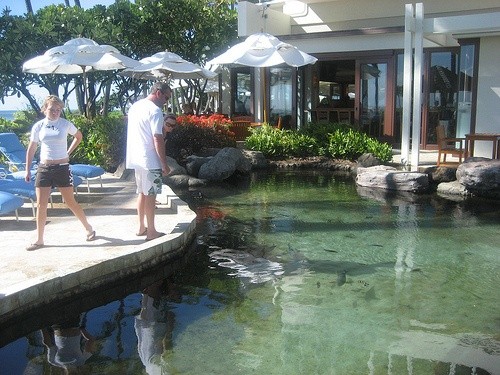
[250,208,422,289]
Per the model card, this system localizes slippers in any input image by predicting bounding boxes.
[26,243,45,251]
[136,227,147,235]
[145,232,166,241]
[86,231,95,241]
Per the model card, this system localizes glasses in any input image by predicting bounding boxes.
[163,94,169,100]
[164,120,176,129]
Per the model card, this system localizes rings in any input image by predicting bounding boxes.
[167,172,168,173]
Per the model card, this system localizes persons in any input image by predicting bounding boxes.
[40,315,96,375]
[25,95,96,251]
[126,81,172,241]
[134,278,174,375]
[181,102,198,114]
[154,115,176,208]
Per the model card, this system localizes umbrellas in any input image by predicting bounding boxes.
[206,32,318,123]
[21,36,143,116]
[422,65,461,93]
[120,49,218,83]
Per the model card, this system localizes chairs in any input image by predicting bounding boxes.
[435,125,470,167]
[0,133,106,223]
[272,113,282,129]
[316,108,440,143]
[232,116,253,124]
[231,120,251,141]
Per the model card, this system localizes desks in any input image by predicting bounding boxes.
[251,122,264,127]
[308,108,440,138]
[464,133,500,160]
